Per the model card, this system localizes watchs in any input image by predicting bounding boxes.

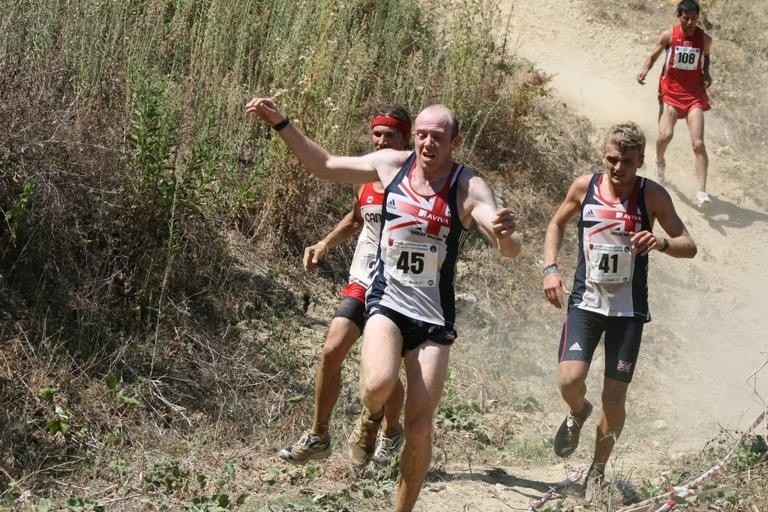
[658,238,669,252]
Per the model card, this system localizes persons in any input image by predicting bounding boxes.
[542,120,697,504]
[278,103,413,467]
[245,96,522,512]
[635,0,713,208]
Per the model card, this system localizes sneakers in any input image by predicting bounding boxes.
[654,156,665,184]
[583,476,605,503]
[374,425,404,465]
[697,191,712,208]
[279,429,331,464]
[554,398,593,458]
[349,403,384,468]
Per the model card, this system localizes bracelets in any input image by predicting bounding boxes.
[542,263,559,277]
[272,116,290,132]
[318,241,329,254]
